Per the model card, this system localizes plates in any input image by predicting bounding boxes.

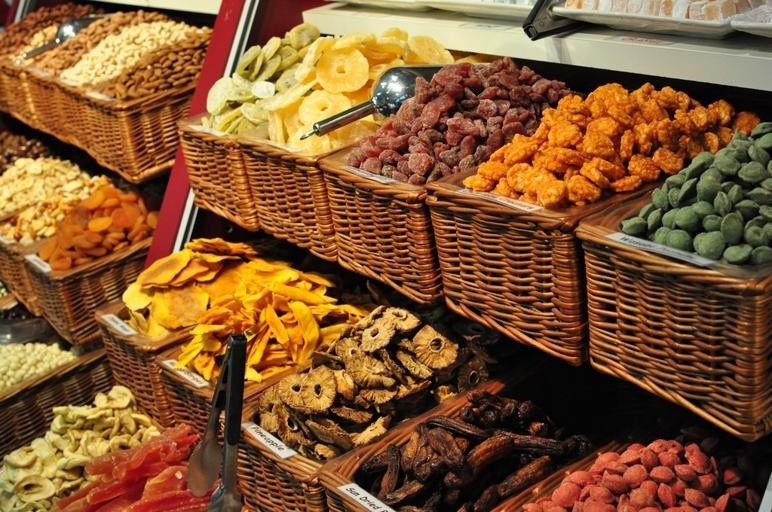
[422,1,534,24]
[551,4,746,40]
[331,1,425,12]
[728,10,772,34]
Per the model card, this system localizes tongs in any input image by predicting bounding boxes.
[186,332,248,511]
[522,0,585,40]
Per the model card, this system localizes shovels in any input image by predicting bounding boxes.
[22,18,101,60]
[300,64,440,140]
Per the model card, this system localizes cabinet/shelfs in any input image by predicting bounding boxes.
[10,0,772,306]
